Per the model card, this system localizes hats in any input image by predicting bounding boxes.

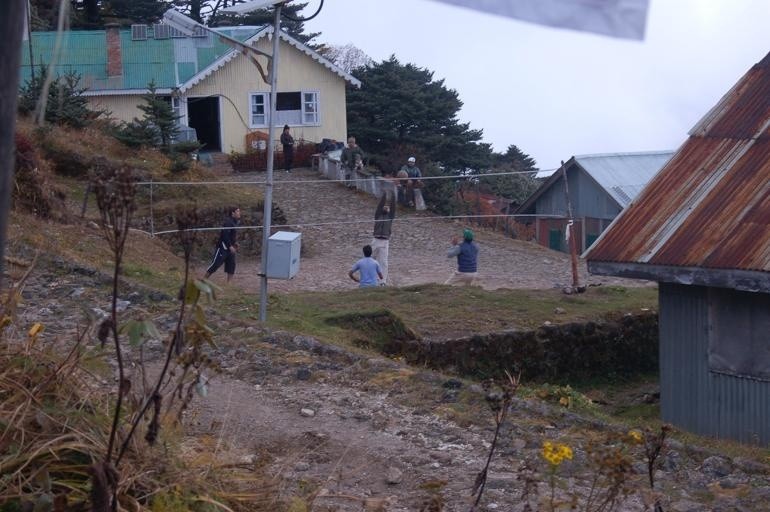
[284,125,289,128]
[348,137,355,143]
[462,227,474,241]
[408,157,415,163]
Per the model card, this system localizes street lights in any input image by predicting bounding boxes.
[164,9,282,322]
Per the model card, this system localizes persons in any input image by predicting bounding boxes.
[280,124,294,173]
[398,157,422,207]
[347,245,384,288]
[354,154,364,170]
[370,188,396,286]
[203,207,241,286]
[319,139,338,157]
[341,136,364,188]
[444,227,480,286]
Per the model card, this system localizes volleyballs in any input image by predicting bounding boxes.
[396,170,409,183]
